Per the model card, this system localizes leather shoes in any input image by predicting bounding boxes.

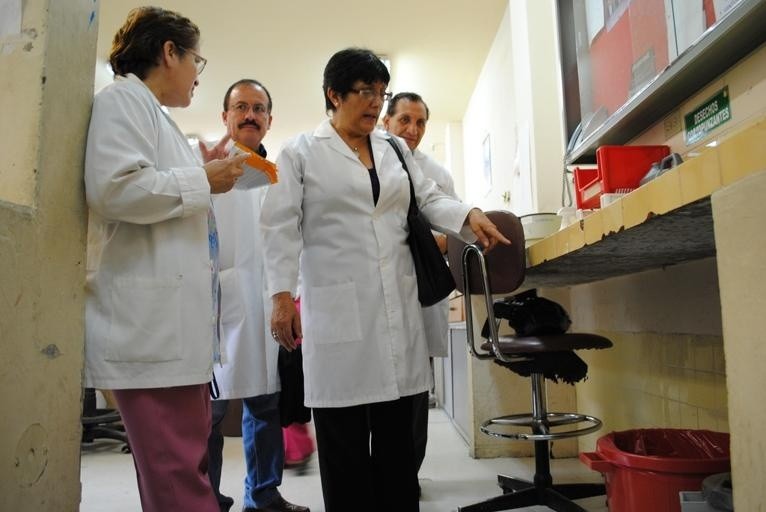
[241,496,310,512]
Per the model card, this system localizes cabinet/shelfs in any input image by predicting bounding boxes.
[433,286,576,458]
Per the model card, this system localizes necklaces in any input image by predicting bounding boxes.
[335,128,369,153]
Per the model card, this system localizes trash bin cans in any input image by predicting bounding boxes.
[680,472,734,512]
[579,428,733,512]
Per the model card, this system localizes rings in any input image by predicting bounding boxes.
[271,330,280,343]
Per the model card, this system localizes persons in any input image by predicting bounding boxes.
[262,48,513,508]
[374,92,467,362]
[197,80,312,511]
[80,7,254,512]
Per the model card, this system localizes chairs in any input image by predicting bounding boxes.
[446,208,606,511]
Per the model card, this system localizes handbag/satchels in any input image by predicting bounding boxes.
[387,135,456,307]
[481,288,571,338]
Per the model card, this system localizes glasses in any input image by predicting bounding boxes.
[349,87,391,101]
[177,44,206,74]
